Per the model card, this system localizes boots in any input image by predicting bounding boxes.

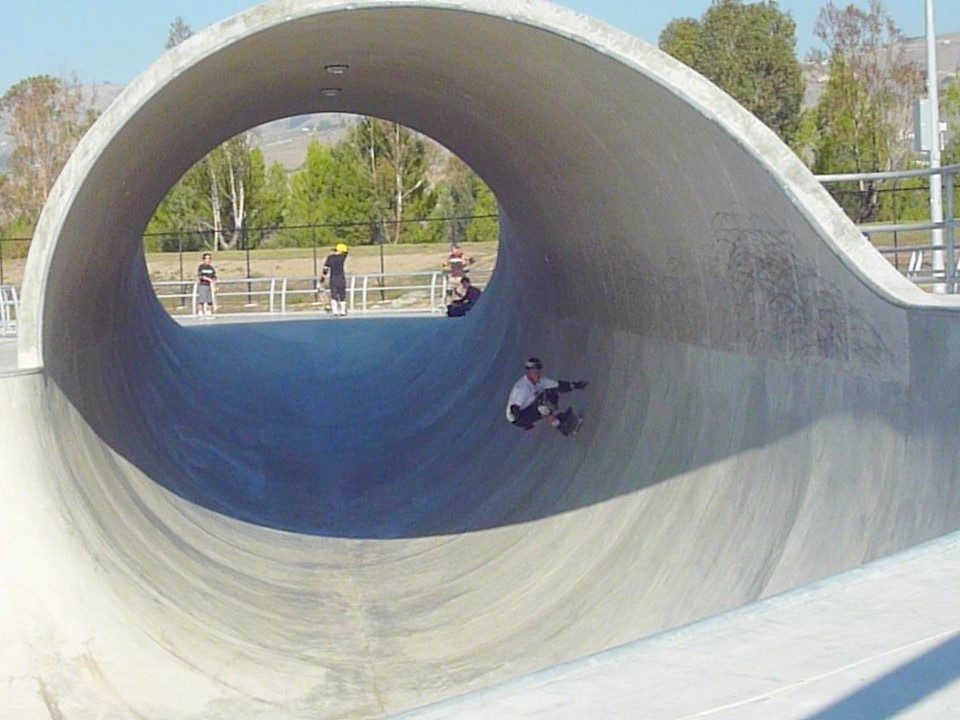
[330,299,337,316]
[339,301,346,316]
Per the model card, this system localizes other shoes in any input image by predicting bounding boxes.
[557,405,572,435]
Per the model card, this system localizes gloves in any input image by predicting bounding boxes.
[537,406,551,416]
[573,381,587,390]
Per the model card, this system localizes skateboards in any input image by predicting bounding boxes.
[569,415,584,436]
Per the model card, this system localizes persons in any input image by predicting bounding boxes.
[197,254,218,322]
[441,244,482,318]
[506,358,588,439]
[317,243,351,317]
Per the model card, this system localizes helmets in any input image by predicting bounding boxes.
[335,243,347,253]
[525,358,542,370]
